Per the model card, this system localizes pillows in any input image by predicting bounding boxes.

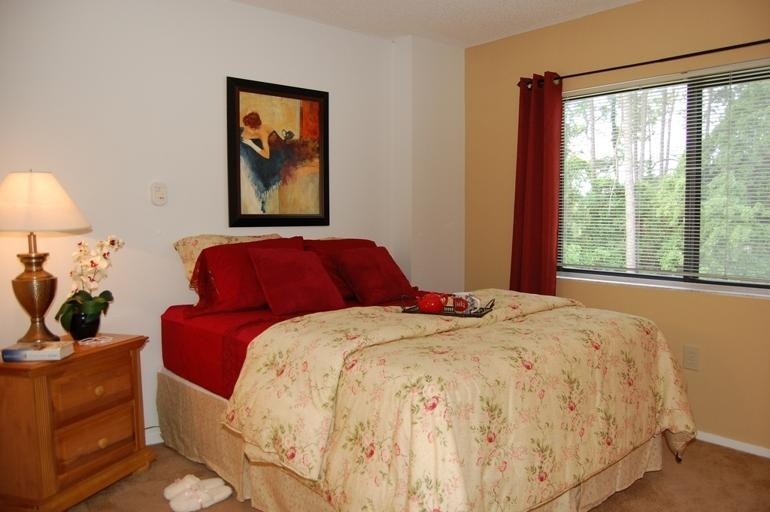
[171,232,416,317]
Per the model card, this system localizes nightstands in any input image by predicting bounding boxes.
[0,331,150,510]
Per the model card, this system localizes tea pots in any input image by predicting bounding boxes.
[414,290,449,313]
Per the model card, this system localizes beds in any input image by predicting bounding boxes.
[156,291,664,512]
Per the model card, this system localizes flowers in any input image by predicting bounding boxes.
[54,234,125,332]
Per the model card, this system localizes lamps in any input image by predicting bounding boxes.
[0,166,92,342]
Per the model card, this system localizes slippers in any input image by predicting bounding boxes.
[169,486,233,512]
[163,473,225,500]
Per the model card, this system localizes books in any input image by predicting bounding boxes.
[1,339,77,363]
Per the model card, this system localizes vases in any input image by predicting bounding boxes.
[70,312,100,340]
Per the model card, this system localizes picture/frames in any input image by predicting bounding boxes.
[225,74,332,229]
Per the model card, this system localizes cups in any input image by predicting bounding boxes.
[450,292,481,315]
